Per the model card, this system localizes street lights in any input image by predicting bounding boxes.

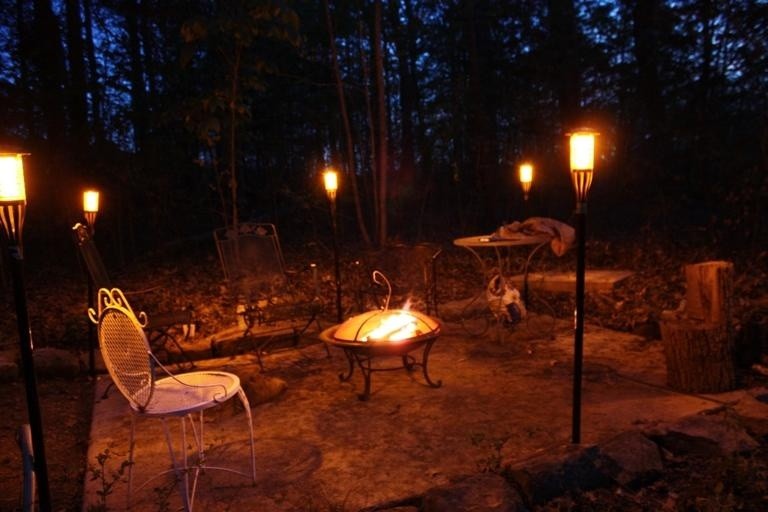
[518,162,535,220]
[0,151,52,512]
[83,189,100,372]
[564,126,601,442]
[321,168,343,323]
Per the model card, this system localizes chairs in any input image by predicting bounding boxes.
[209,222,337,372]
[86,286,260,510]
[658,260,739,396]
[346,242,444,319]
[70,221,201,400]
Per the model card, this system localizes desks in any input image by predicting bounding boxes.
[452,235,557,342]
